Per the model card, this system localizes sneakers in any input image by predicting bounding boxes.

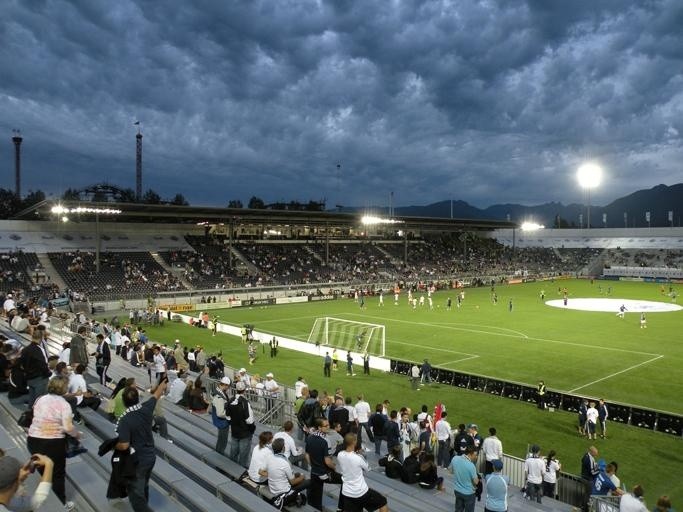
[64,500,74,512]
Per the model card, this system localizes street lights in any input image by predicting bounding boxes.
[337,164,342,208]
[447,192,454,218]
[389,191,395,215]
[11,128,24,198]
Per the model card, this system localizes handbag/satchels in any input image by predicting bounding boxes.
[17,411,34,428]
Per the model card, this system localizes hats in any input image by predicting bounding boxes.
[220,376,230,385]
[236,382,245,391]
[266,372,274,378]
[238,368,246,373]
[0,455,20,489]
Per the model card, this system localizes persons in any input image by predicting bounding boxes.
[0,212,682,512]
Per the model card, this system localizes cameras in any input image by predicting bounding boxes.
[30,459,36,473]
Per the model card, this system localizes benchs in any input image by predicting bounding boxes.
[0,234,681,512]
